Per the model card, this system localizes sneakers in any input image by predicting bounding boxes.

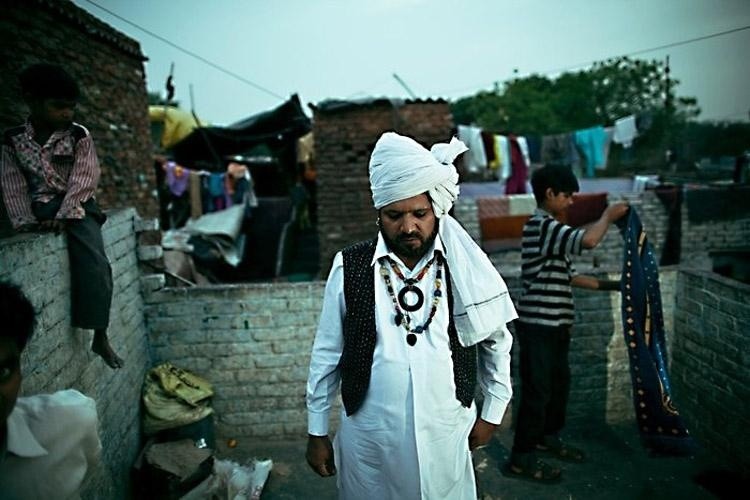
[535,442,555,455]
[503,457,560,484]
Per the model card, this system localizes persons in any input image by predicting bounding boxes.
[1,280,104,500]
[500,164,629,486]
[290,175,312,237]
[665,143,679,172]
[296,130,318,236]
[1,62,125,369]
[305,132,520,500]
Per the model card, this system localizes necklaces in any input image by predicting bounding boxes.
[385,256,437,312]
[378,257,443,346]
